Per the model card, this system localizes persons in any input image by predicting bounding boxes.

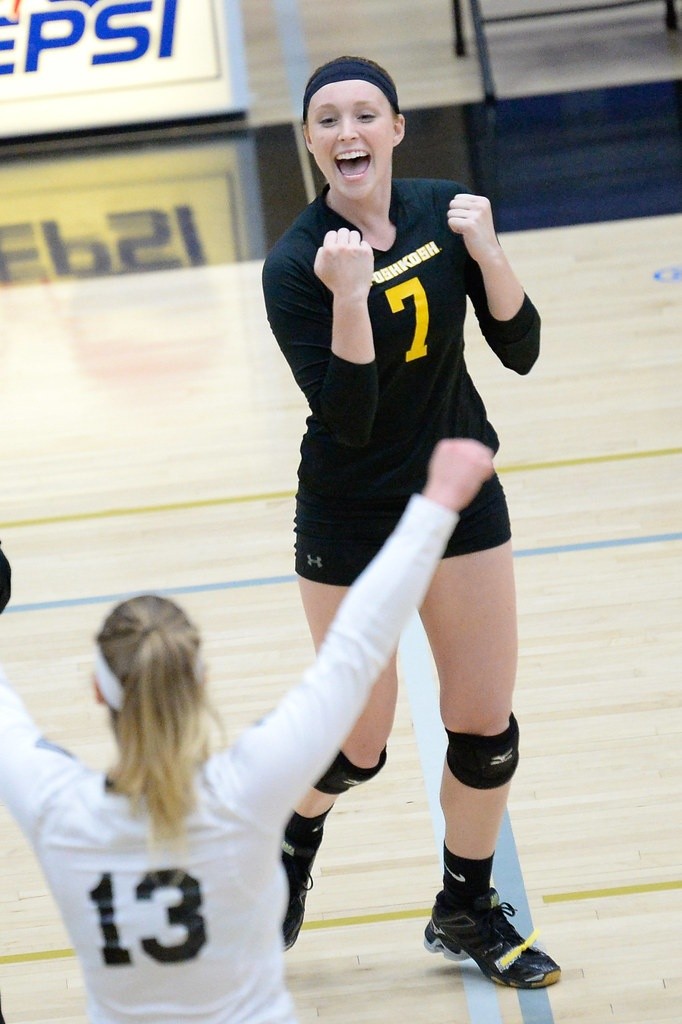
[0,437,498,1024]
[260,57,566,995]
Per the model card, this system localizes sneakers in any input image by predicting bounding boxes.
[280,841,314,951]
[423,887,562,989]
